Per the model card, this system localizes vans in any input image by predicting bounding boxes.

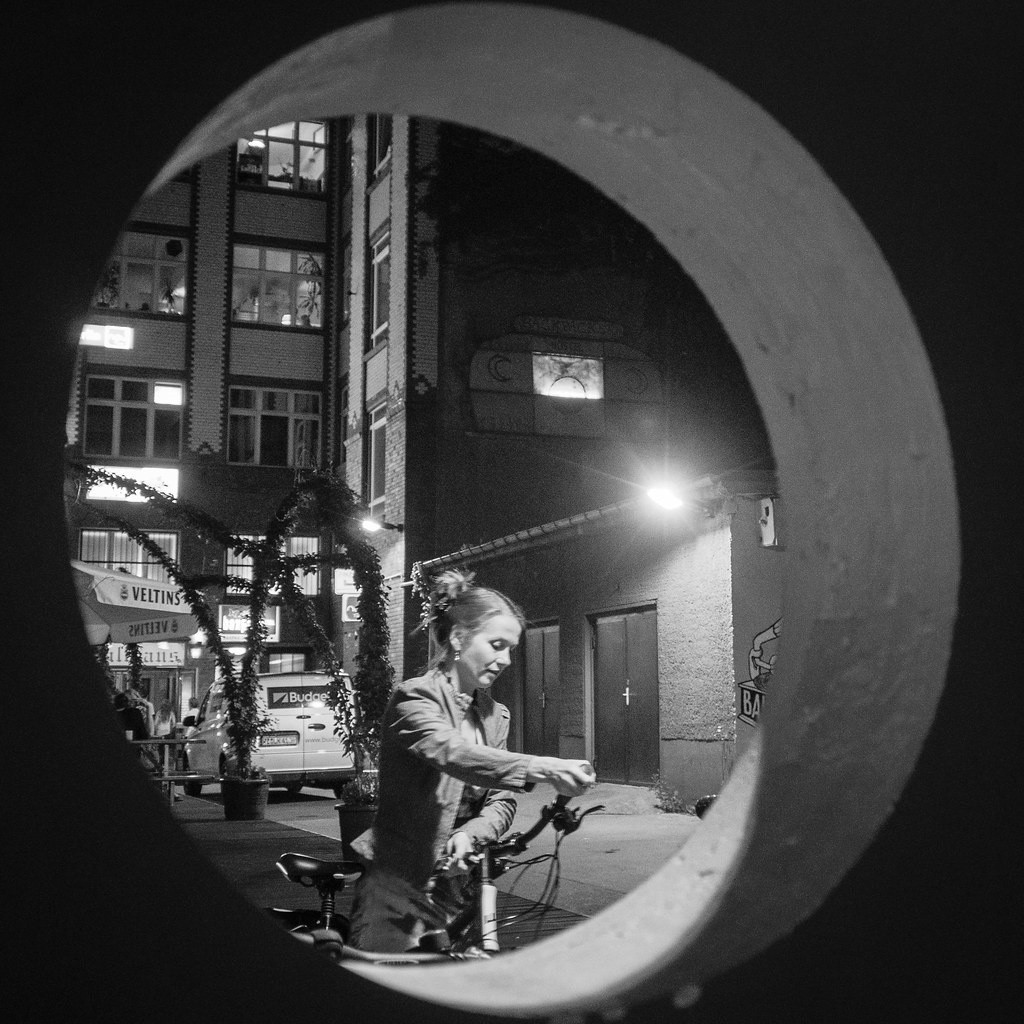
[181,669,359,804]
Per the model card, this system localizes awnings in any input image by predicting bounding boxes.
[74,557,206,646]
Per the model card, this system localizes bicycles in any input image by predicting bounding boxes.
[265,764,605,968]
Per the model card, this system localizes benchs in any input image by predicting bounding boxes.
[151,774,215,806]
[148,771,198,797]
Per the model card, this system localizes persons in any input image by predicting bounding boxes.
[347,585,597,953]
[181,696,200,771]
[113,684,155,792]
[154,699,177,777]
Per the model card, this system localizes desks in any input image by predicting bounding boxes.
[127,738,207,797]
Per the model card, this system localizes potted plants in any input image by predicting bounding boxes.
[96,261,119,308]
[61,442,445,904]
